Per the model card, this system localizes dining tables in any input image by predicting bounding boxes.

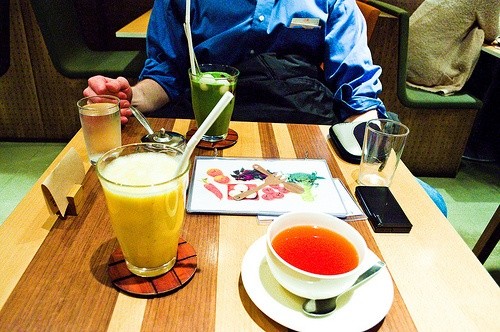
[0,117,500,332]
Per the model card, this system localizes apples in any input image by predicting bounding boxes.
[207,168,229,184]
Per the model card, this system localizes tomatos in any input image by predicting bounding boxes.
[262,184,288,200]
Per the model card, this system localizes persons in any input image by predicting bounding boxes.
[82,0,448,219]
[406,0,500,163]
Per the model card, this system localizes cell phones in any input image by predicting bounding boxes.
[355,186,412,233]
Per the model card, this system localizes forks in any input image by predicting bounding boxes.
[233,172,287,201]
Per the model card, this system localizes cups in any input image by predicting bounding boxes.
[188,63,240,142]
[97,143,192,278]
[77,94,122,167]
[357,118,410,187]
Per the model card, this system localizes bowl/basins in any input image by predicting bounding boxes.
[265,210,367,299]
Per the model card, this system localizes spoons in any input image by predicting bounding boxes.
[252,163,305,194]
[301,260,386,316]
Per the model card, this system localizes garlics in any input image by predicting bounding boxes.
[229,184,257,198]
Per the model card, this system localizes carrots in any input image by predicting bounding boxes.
[202,179,223,200]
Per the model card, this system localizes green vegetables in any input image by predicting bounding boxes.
[286,171,325,202]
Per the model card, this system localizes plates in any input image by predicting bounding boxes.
[240,232,394,332]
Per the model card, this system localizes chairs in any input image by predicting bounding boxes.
[19,0,140,141]
[372,0,478,179]
[0,2,46,140]
